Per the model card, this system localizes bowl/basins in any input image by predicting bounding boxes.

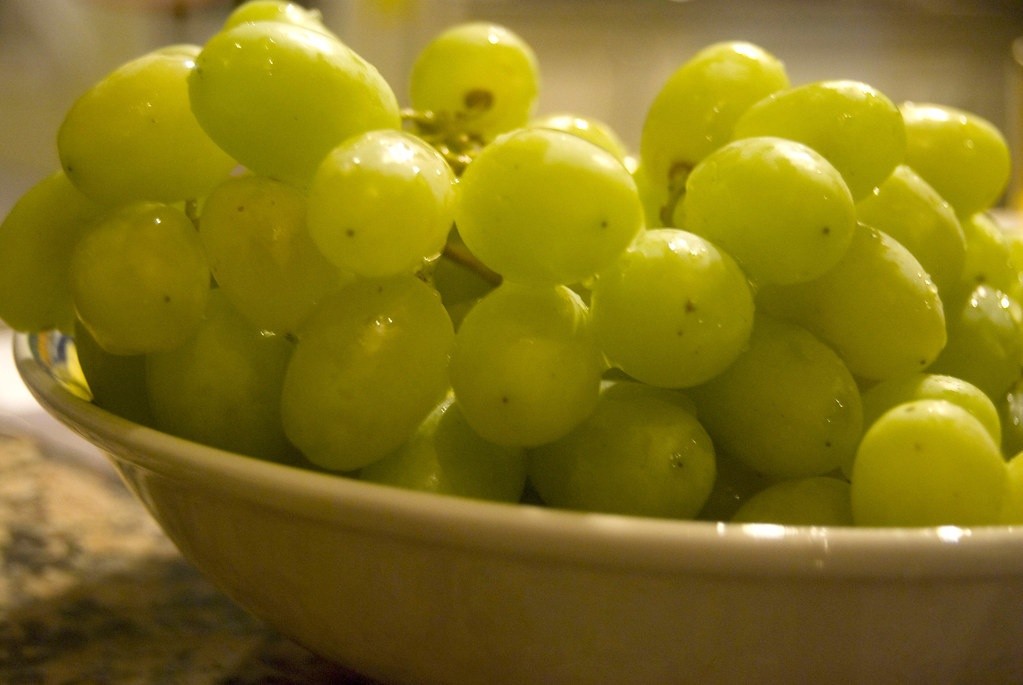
[10,311,1023,685]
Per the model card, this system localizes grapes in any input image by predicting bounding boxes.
[0,0,1023,527]
